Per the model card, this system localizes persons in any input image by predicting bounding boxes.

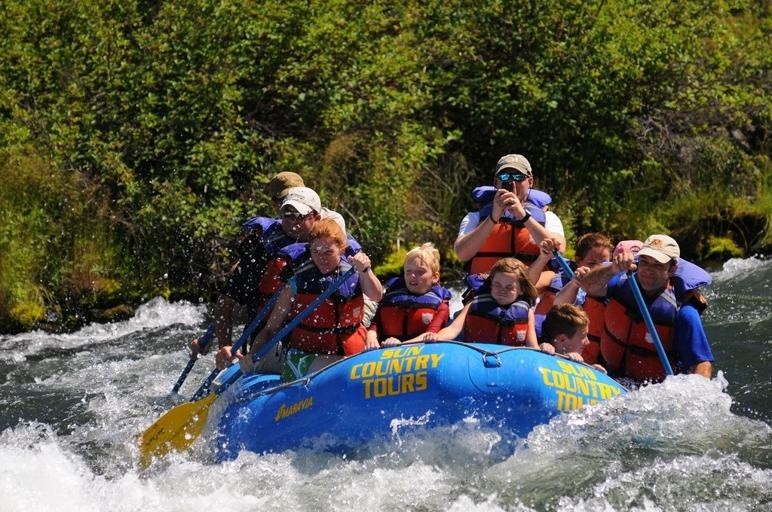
[364,242,451,350]
[455,154,567,319]
[189,172,384,382]
[423,257,555,355]
[529,234,715,391]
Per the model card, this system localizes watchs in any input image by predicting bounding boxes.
[517,208,531,223]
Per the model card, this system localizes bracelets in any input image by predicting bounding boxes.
[490,213,498,224]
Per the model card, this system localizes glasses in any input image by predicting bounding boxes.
[498,172,525,181]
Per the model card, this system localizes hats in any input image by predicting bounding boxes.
[614,239,642,257]
[271,171,304,198]
[637,234,680,264]
[495,154,532,176]
[280,187,321,216]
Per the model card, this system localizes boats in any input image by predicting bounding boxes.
[197,341,628,464]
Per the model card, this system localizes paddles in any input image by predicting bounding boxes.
[137,245,369,467]
